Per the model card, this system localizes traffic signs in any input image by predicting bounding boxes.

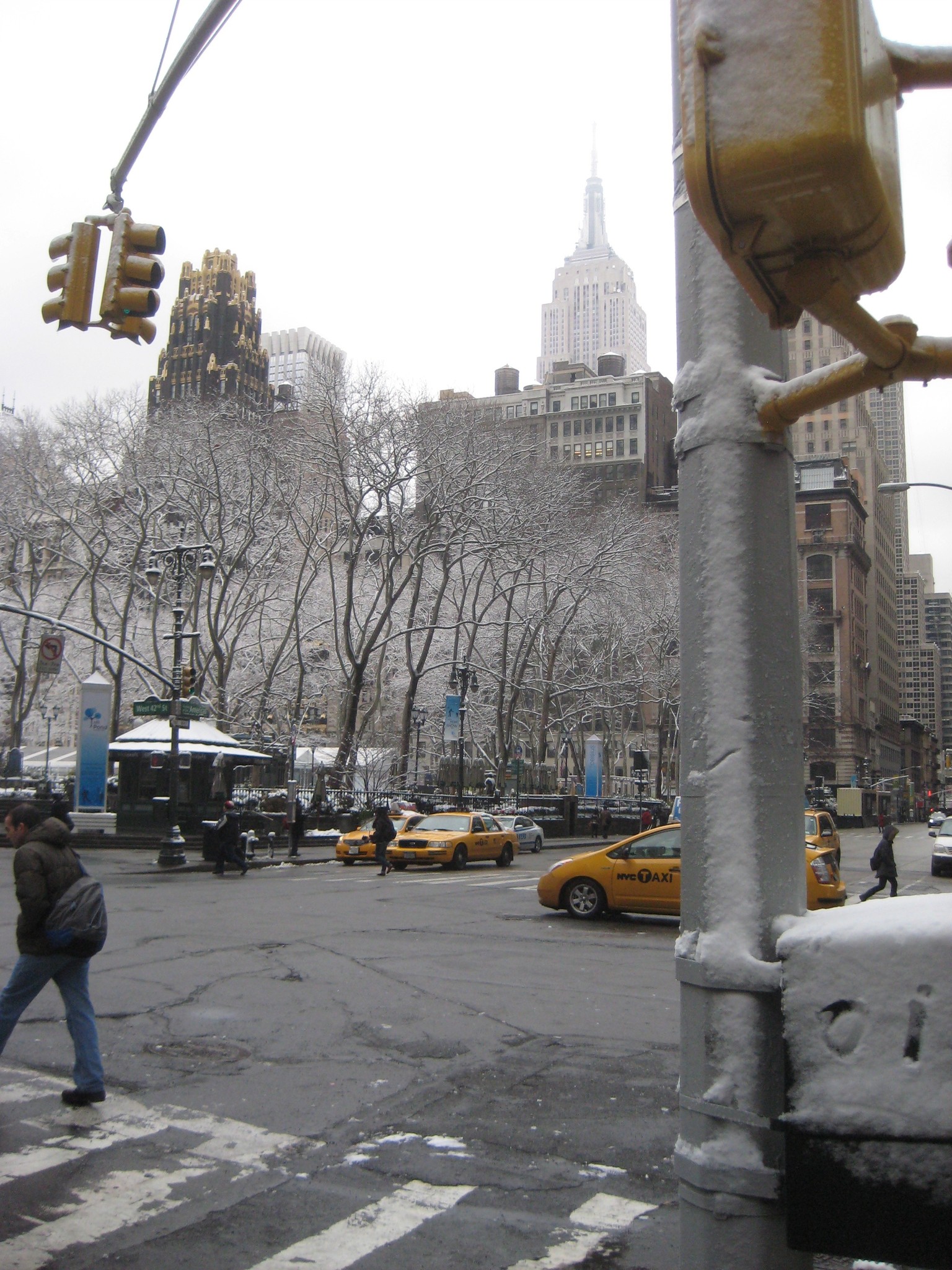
[168,715,189,730]
[179,697,210,718]
[133,695,171,717]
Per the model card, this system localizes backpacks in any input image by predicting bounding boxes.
[380,817,397,840]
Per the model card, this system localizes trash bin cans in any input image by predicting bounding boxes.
[200,819,223,861]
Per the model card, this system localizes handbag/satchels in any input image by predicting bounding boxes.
[64,815,75,831]
[42,876,108,961]
[870,857,880,870]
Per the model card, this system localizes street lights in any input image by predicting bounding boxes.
[146,525,217,864]
[39,700,62,781]
[310,744,318,789]
[447,650,480,811]
[560,729,573,795]
[411,705,429,786]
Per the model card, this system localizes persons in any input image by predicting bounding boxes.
[483,772,500,797]
[358,794,438,816]
[1,804,108,1106]
[652,806,673,829]
[857,825,899,901]
[590,809,600,837]
[285,805,303,856]
[878,812,884,834]
[895,807,932,825]
[62,775,121,814]
[364,807,395,876]
[208,800,250,875]
[44,793,69,834]
[641,809,652,832]
[601,805,611,839]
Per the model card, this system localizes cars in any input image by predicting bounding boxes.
[492,815,545,853]
[336,814,430,870]
[537,821,846,920]
[385,810,521,871]
[928,812,946,828]
[805,810,841,872]
[928,814,952,876]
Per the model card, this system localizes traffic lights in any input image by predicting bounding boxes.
[928,790,931,796]
[183,666,197,699]
[149,750,166,769]
[178,751,192,769]
[97,211,166,326]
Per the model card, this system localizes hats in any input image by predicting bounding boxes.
[376,806,388,814]
[225,800,234,809]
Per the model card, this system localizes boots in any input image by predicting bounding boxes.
[378,866,386,876]
[387,862,393,874]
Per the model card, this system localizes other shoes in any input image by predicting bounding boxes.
[241,864,250,875]
[62,1088,106,1105]
[859,895,866,902]
[212,868,224,874]
